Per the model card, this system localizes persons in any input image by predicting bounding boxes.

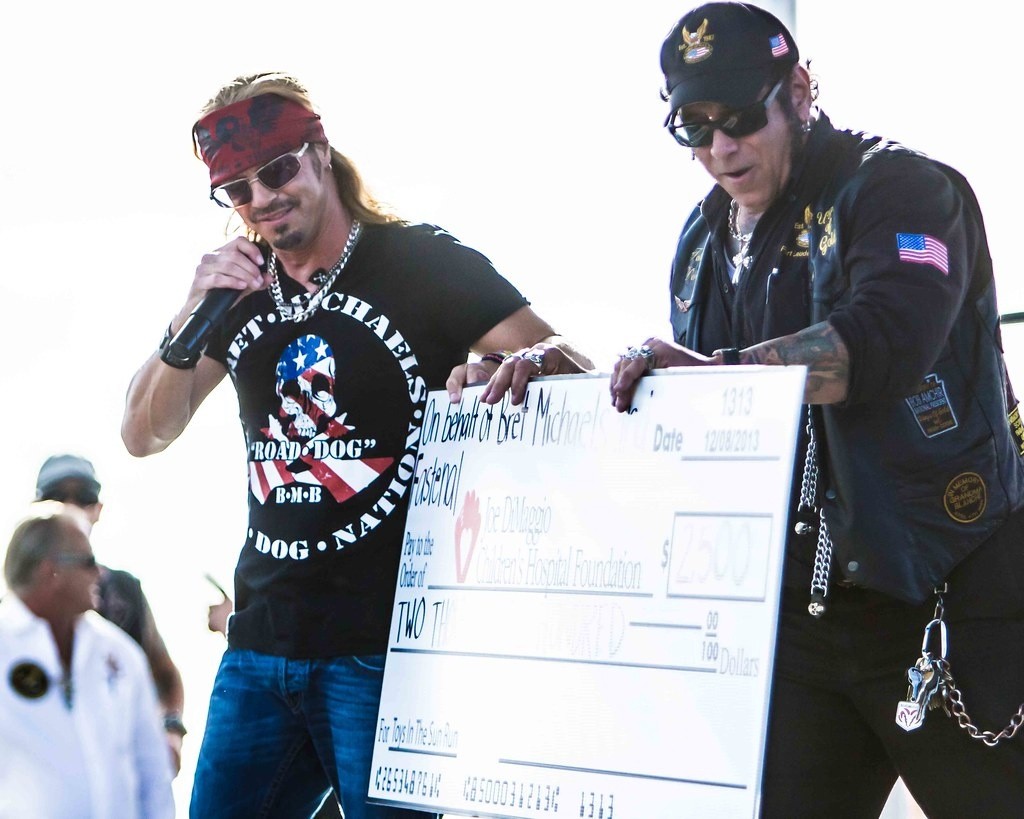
[121,71,595,819]
[0,455,186,819]
[609,2,1023,819]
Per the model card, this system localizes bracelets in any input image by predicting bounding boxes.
[162,715,187,736]
[482,350,504,363]
[712,348,739,364]
[159,322,201,369]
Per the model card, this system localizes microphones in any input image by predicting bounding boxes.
[169,241,269,361]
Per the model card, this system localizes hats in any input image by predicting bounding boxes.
[36,455,103,497]
[661,4,797,129]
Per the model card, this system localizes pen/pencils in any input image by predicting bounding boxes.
[765,268,779,304]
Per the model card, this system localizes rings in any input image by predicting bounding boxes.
[619,345,655,370]
[502,346,545,370]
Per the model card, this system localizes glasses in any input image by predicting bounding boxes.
[50,554,98,573]
[34,489,97,508]
[667,78,786,149]
[208,139,309,210]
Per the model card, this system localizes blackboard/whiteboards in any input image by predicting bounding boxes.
[363,365,811,818]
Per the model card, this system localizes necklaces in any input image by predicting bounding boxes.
[266,218,362,323]
